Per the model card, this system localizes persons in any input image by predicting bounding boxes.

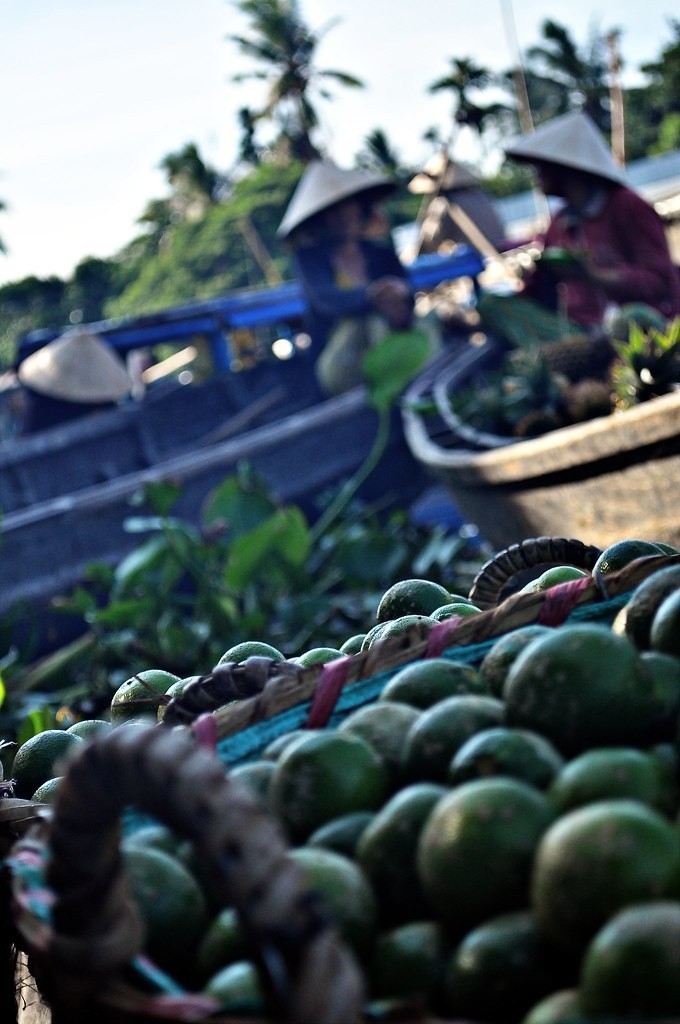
[276,150,507,401]
[505,107,680,341]
[18,326,159,439]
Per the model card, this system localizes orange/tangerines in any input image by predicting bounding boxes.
[9,563,680,1024]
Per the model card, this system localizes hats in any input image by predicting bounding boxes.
[278,157,399,239]
[505,110,629,186]
[407,149,477,194]
[18,333,133,403]
